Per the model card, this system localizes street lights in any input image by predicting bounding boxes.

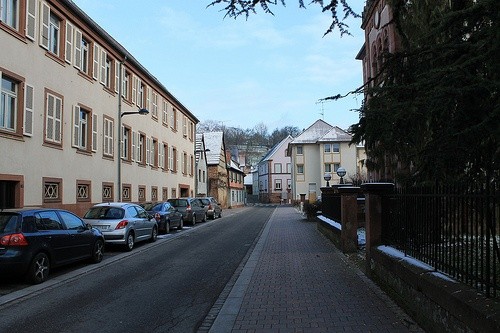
[194,149,211,197]
[118,108,150,202]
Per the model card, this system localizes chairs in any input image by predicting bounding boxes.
[106,209,114,217]
[113,212,122,218]
[38,216,57,230]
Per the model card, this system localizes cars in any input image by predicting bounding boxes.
[82,202,158,250]
[197,197,223,220]
[137,200,185,235]
[0,207,105,284]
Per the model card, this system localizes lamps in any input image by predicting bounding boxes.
[336,167,347,184]
[324,173,332,187]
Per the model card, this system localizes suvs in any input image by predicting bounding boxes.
[166,196,208,226]
